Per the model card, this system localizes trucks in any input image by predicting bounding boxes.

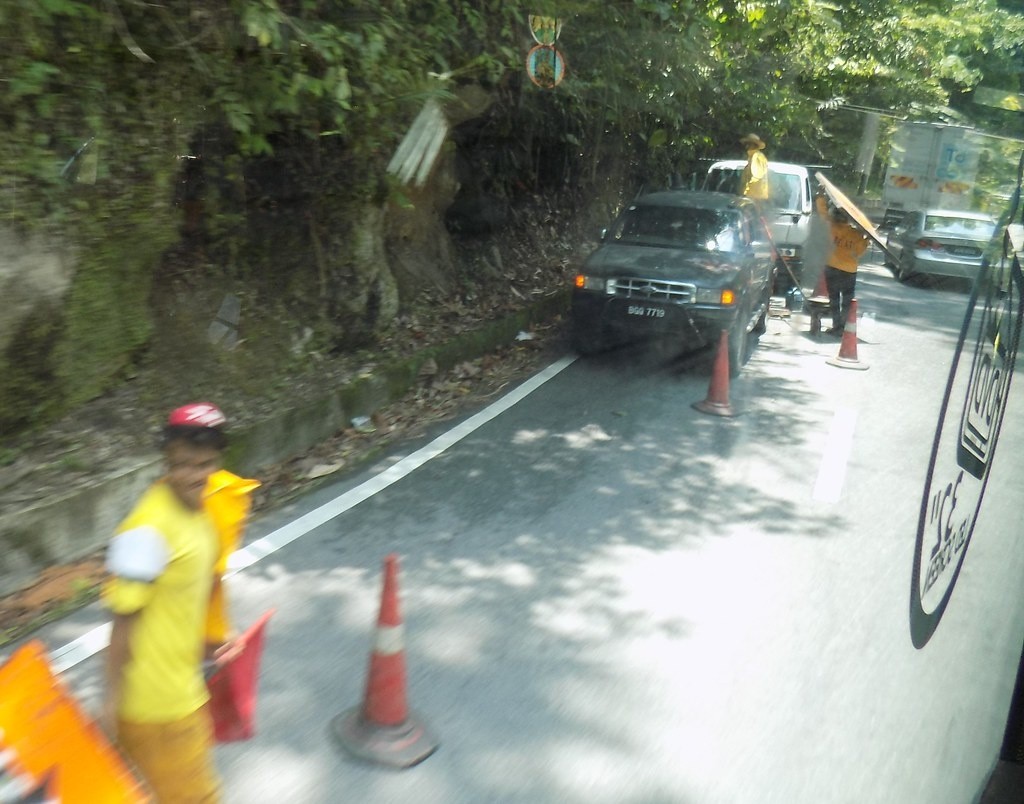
[879,121,989,231]
[700,160,813,285]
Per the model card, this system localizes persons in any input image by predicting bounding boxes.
[736,133,768,200]
[815,185,871,334]
[99,403,229,804]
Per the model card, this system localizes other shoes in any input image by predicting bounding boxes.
[825,327,844,336]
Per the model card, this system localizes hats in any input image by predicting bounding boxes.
[739,133,766,150]
[167,402,229,435]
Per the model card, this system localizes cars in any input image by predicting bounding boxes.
[883,210,998,283]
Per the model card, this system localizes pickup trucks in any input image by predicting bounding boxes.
[571,188,776,378]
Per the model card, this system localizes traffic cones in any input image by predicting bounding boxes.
[827,298,872,371]
[327,554,444,772]
[692,329,742,417]
